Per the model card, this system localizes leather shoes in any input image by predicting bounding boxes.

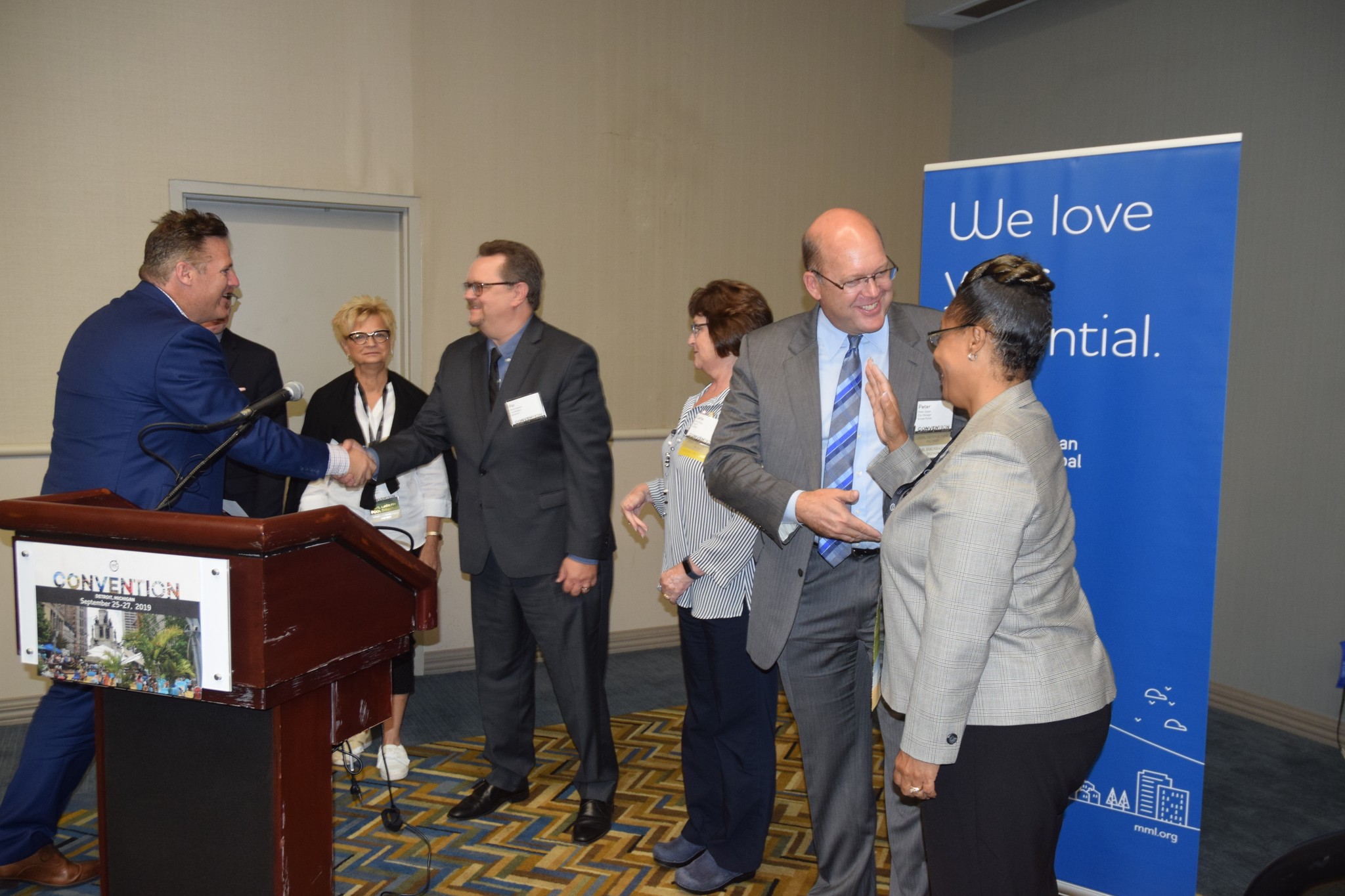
[0,845,99,888]
[447,778,530,820]
[572,798,615,844]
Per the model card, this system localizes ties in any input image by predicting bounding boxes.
[489,347,504,412]
[817,335,865,568]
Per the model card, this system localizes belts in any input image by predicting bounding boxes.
[814,542,882,557]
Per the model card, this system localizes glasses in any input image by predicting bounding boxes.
[226,293,239,307]
[344,329,391,345]
[691,323,707,334]
[812,254,898,294]
[464,282,513,297]
[928,324,991,347]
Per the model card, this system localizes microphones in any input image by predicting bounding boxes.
[231,381,305,424]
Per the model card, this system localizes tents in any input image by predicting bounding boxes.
[43,644,62,653]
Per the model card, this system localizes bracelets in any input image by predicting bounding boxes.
[682,555,701,579]
[425,532,443,541]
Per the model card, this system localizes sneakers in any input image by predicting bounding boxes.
[332,728,372,766]
[378,744,410,780]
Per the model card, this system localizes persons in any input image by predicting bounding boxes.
[866,257,1117,896]
[334,238,618,842]
[0,209,377,890]
[703,213,967,896]
[621,281,776,892]
[201,295,288,519]
[38,646,201,700]
[281,298,452,783]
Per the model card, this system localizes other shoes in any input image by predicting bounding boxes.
[675,850,757,894]
[653,834,706,868]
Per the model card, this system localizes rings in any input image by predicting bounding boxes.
[910,786,919,794]
[628,523,630,525]
[664,594,671,599]
[582,588,590,590]
